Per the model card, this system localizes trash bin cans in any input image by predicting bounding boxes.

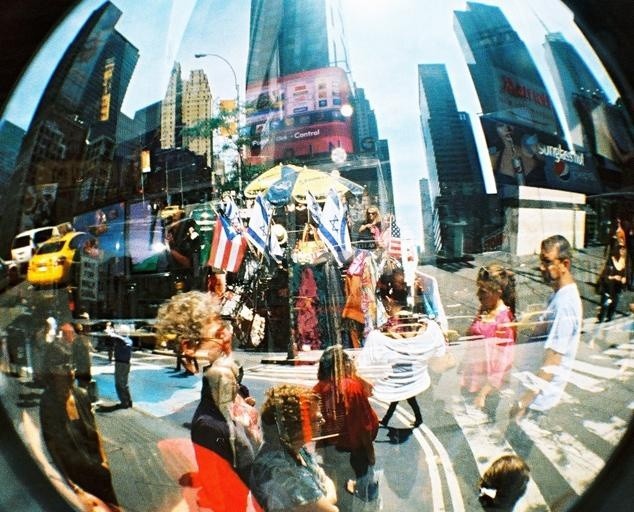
[438,217,469,259]
[433,196,456,220]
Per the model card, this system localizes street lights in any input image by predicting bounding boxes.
[187,48,244,128]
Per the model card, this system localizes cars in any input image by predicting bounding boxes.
[496,307,585,346]
[126,324,176,349]
[0,219,100,293]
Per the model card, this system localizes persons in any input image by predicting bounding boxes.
[601,215,634,260]
[441,251,516,453]
[487,234,581,463]
[24,188,53,228]
[2,290,265,511]
[353,265,445,427]
[307,347,381,511]
[145,196,167,253]
[477,449,530,512]
[246,386,340,512]
[484,121,551,191]
[592,239,628,322]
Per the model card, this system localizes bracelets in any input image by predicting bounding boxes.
[475,390,487,401]
[516,399,525,411]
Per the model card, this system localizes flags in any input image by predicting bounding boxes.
[182,185,400,274]
